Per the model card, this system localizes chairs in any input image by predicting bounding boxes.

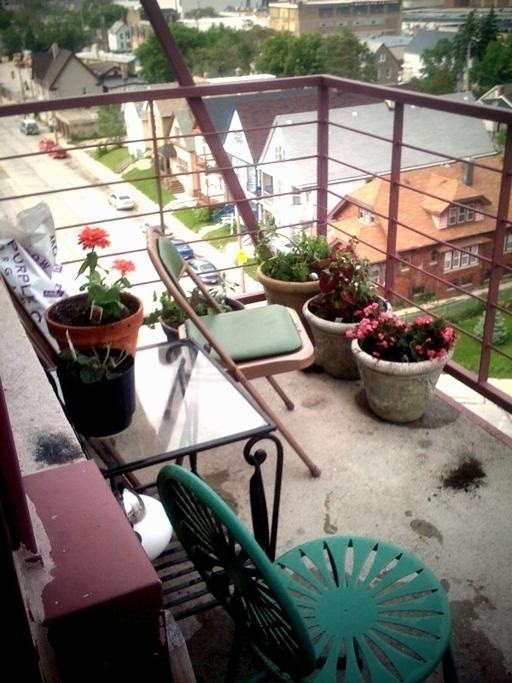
[146,227,322,477]
[156,462,458,682]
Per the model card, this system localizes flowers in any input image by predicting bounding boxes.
[74,226,135,316]
[256,225,458,358]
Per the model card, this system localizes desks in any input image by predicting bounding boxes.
[48,337,284,493]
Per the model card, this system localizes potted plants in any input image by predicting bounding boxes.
[144,269,245,340]
[57,345,136,436]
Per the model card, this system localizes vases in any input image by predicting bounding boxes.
[257,255,453,424]
[44,291,144,360]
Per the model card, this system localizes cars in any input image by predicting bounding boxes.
[108,192,134,211]
[40,139,67,159]
[212,203,267,223]
[143,220,193,259]
[21,120,39,135]
[186,259,220,284]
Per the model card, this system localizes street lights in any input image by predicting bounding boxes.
[102,81,166,235]
